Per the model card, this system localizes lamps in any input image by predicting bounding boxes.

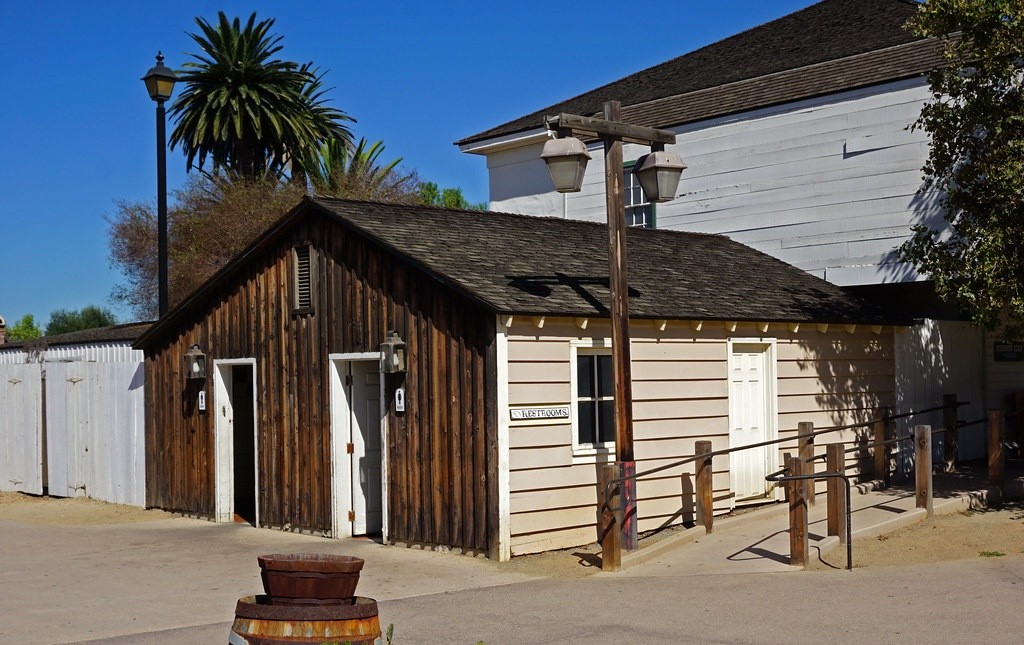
[185,345,206,381]
[379,331,407,375]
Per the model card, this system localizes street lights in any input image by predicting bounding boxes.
[538,97,687,547]
[140,49,182,322]
